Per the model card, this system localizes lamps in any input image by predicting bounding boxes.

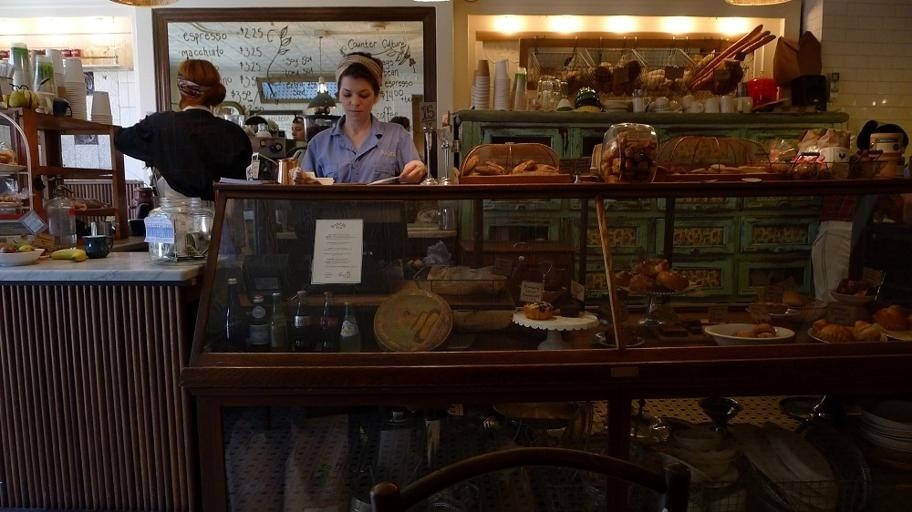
[306,37,337,117]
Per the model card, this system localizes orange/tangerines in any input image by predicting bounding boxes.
[795,162,817,178]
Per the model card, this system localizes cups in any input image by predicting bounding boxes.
[438,207,458,230]
[278,158,299,185]
[217,114,244,128]
[536,75,603,115]
[91,91,113,126]
[790,73,828,113]
[1,42,88,122]
[819,147,850,179]
[746,78,776,106]
[83,235,114,259]
[470,58,528,113]
[605,93,753,114]
[91,221,113,252]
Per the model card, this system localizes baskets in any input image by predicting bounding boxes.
[528,46,755,112]
[414,265,510,297]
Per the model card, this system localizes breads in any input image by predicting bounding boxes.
[615,257,688,292]
[464,156,555,175]
[692,164,764,174]
[812,304,909,342]
[737,324,776,338]
[782,291,810,306]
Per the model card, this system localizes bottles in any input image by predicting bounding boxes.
[224,277,361,353]
[47,184,79,252]
[147,197,214,265]
[894,153,904,177]
[255,123,271,138]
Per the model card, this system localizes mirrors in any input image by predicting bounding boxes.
[151,6,436,160]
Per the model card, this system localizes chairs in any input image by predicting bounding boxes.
[369,445,690,512]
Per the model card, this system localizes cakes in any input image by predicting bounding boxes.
[524,302,553,319]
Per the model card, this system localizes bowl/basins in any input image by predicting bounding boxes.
[298,114,344,143]
[704,322,795,344]
[129,220,146,236]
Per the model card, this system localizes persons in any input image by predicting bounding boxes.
[290,51,429,263]
[808,119,910,304]
[291,116,305,140]
[244,116,268,133]
[390,116,411,132]
[114,58,254,260]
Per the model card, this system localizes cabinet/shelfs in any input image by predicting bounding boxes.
[454,110,849,308]
[0,106,129,241]
[178,174,912,511]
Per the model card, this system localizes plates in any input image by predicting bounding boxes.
[668,397,910,511]
[1,201,26,207]
[806,324,888,345]
[1,248,46,265]
[830,291,880,306]
[0,163,28,171]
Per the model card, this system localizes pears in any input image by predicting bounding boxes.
[24,90,40,110]
[9,90,26,107]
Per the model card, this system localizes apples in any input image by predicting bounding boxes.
[0,239,34,253]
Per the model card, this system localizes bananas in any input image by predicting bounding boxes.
[52,248,87,262]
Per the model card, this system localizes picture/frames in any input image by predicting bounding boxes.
[250,110,304,141]
